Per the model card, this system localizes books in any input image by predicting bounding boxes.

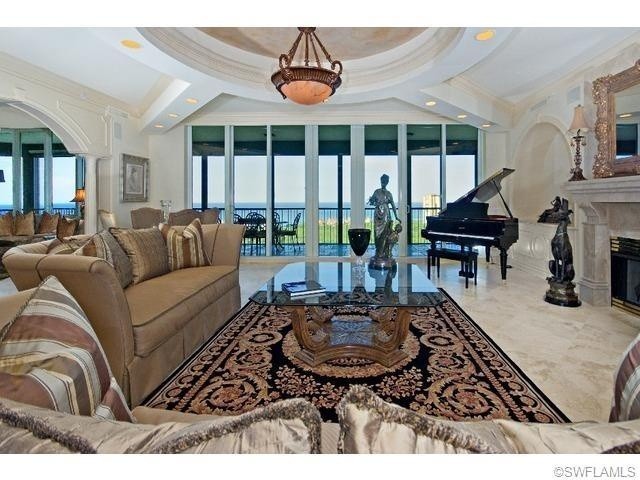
[282,279,326,298]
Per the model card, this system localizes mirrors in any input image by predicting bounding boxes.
[589,58,640,176]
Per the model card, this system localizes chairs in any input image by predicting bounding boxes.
[232,210,303,257]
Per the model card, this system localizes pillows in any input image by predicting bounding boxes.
[0,206,216,285]
[0,273,135,424]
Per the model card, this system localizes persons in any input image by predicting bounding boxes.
[386,219,402,258]
[537,196,561,222]
[368,174,401,260]
[129,168,141,192]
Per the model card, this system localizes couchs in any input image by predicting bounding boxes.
[4,218,247,412]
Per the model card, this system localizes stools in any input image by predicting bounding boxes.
[427,248,479,288]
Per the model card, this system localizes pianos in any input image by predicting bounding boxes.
[420,168,519,280]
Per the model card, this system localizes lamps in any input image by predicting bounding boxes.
[70,186,86,218]
[269,28,345,108]
[566,102,592,183]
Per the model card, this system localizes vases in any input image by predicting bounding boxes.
[347,227,373,260]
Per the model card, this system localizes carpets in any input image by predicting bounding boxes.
[138,280,574,427]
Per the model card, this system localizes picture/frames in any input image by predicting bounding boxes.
[118,152,151,203]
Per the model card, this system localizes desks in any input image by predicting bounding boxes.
[248,250,444,370]
